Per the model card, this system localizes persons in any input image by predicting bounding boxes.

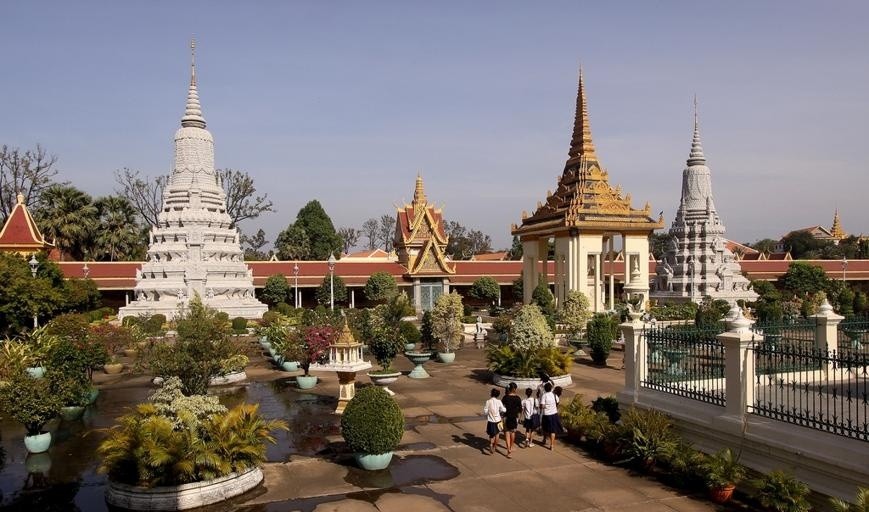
[483,374,563,457]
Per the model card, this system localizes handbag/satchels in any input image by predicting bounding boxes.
[518,413,524,424]
[532,414,540,427]
[497,422,504,431]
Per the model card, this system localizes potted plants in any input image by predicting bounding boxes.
[556,393,705,482]
[484,302,576,392]
[2,354,81,454]
[0,287,466,420]
[459,286,752,369]
[340,384,406,470]
[82,375,291,509]
[701,447,745,505]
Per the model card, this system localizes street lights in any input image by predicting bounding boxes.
[840,255,848,282]
[326,249,337,312]
[291,260,299,309]
[81,261,90,279]
[26,252,41,331]
